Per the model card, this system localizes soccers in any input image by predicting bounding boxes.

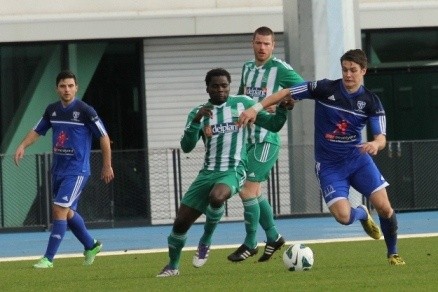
[283,244,314,271]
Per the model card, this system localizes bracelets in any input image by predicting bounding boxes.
[251,103,262,114]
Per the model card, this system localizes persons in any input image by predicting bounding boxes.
[235,49,405,268]
[156,67,289,277]
[226,27,306,264]
[14,71,115,268]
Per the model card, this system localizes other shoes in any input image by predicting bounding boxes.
[84,240,104,264]
[31,258,54,270]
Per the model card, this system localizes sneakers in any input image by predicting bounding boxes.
[156,265,180,278]
[192,242,210,268]
[389,253,407,266]
[356,204,382,241]
[227,242,259,262]
[257,235,286,261]
